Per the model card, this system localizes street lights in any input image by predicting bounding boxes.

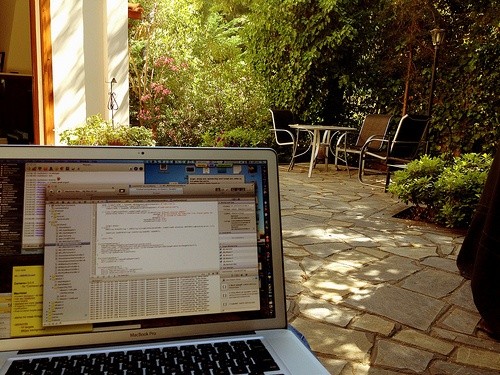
[424,28,446,155]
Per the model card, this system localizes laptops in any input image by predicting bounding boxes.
[0,144,333,375]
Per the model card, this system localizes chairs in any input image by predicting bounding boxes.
[268,108,433,194]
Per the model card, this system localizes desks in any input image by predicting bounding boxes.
[287,123,357,178]
